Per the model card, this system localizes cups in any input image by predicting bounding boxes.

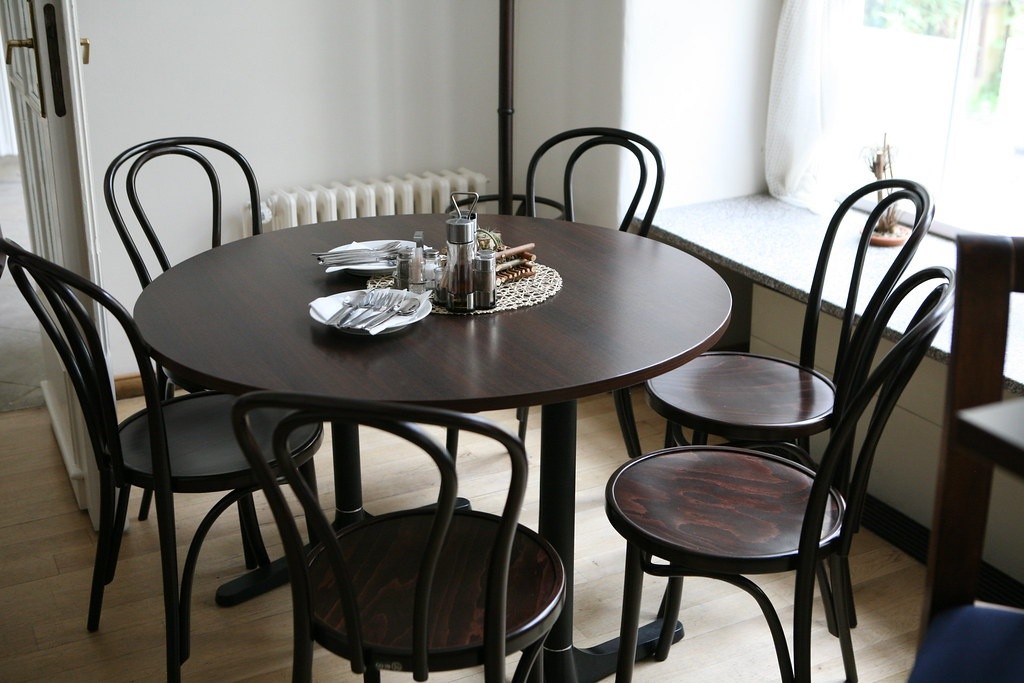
[477,250,497,306]
[474,257,493,310]
[445,211,478,311]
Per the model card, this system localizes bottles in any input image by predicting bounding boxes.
[396,252,412,290]
[433,268,444,302]
[422,249,438,290]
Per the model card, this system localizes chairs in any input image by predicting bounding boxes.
[232,393,569,683]
[518,123,664,463]
[603,266,956,683]
[645,177,936,632]
[101,130,326,524]
[0,229,322,683]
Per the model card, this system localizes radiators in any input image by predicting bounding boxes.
[247,165,492,234]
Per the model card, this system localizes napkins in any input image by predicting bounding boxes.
[318,239,432,275]
[306,289,432,336]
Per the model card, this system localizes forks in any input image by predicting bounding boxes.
[311,242,403,267]
[342,293,403,328]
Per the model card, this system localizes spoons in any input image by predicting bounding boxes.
[370,298,419,328]
[325,291,366,324]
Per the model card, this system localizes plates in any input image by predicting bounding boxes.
[310,289,432,335]
[328,240,416,275]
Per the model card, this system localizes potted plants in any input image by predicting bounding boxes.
[862,134,914,247]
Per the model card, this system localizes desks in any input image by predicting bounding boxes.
[136,213,732,683]
